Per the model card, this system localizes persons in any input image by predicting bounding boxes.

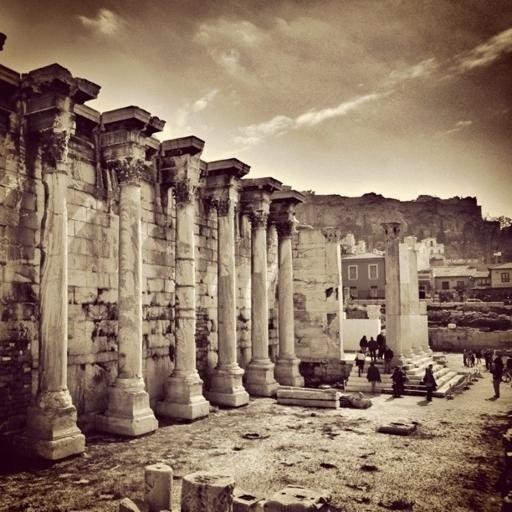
[355,350,365,377]
[368,336,377,362]
[359,335,368,359]
[384,348,393,374]
[376,331,385,360]
[425,364,433,374]
[422,369,437,402]
[463,347,511,399]
[391,366,404,399]
[367,361,382,396]
